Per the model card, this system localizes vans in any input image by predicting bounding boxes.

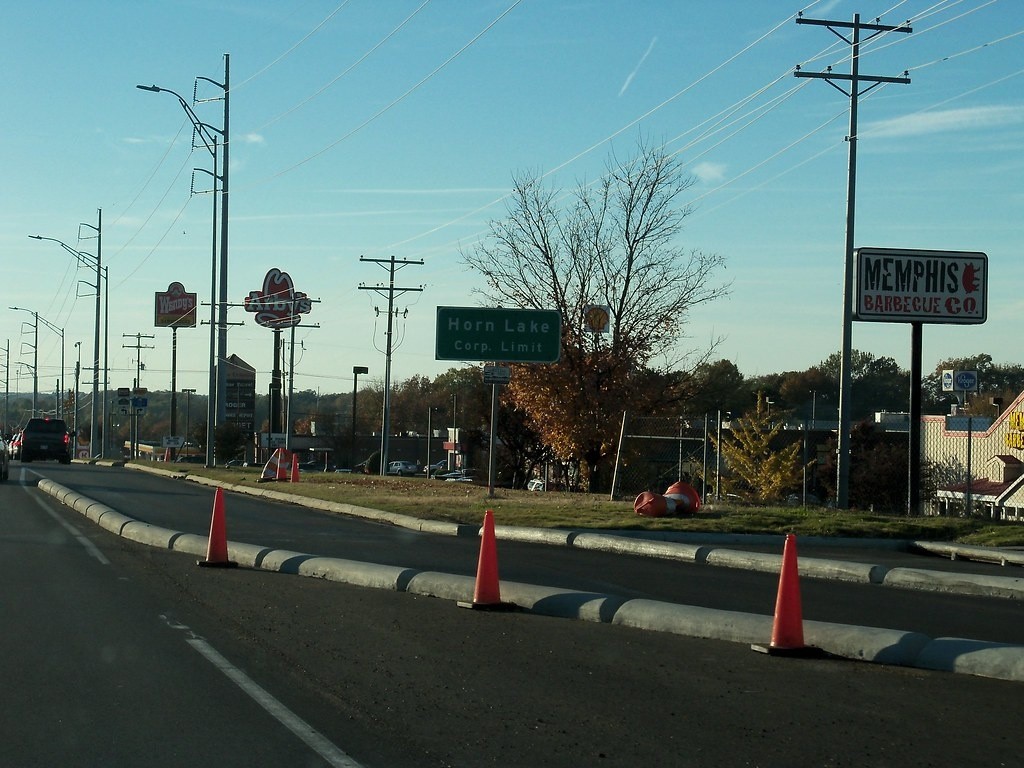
[176,456,202,464]
[226,460,244,469]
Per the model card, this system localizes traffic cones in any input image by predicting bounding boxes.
[456,509,517,611]
[196,486,239,567]
[290,453,300,482]
[750,533,825,656]
[163,447,170,462]
[272,447,290,482]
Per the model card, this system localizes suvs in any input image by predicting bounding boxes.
[21,418,71,463]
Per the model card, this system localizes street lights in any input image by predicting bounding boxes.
[352,367,368,436]
[10,306,64,418]
[182,389,196,454]
[28,235,108,459]
[136,84,216,468]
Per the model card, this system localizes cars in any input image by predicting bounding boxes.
[387,461,418,476]
[300,460,336,471]
[9,433,21,460]
[352,460,368,474]
[423,460,448,475]
[0,430,9,481]
